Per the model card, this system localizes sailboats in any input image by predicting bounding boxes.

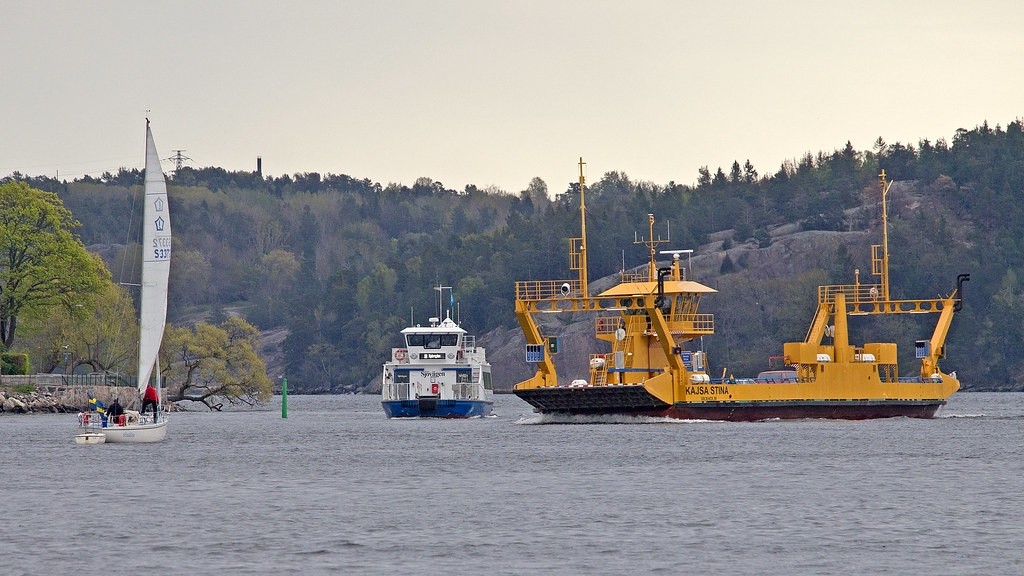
[74,108,174,444]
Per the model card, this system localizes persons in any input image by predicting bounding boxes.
[106,398,123,422]
[141,385,159,423]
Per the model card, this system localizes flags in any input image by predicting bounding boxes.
[89,394,107,413]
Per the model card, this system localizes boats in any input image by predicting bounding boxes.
[381,269,494,418]
[512,157,970,422]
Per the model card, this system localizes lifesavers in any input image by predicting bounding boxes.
[598,319,603,331]
[118,414,127,426]
[83,412,89,424]
[395,348,406,361]
